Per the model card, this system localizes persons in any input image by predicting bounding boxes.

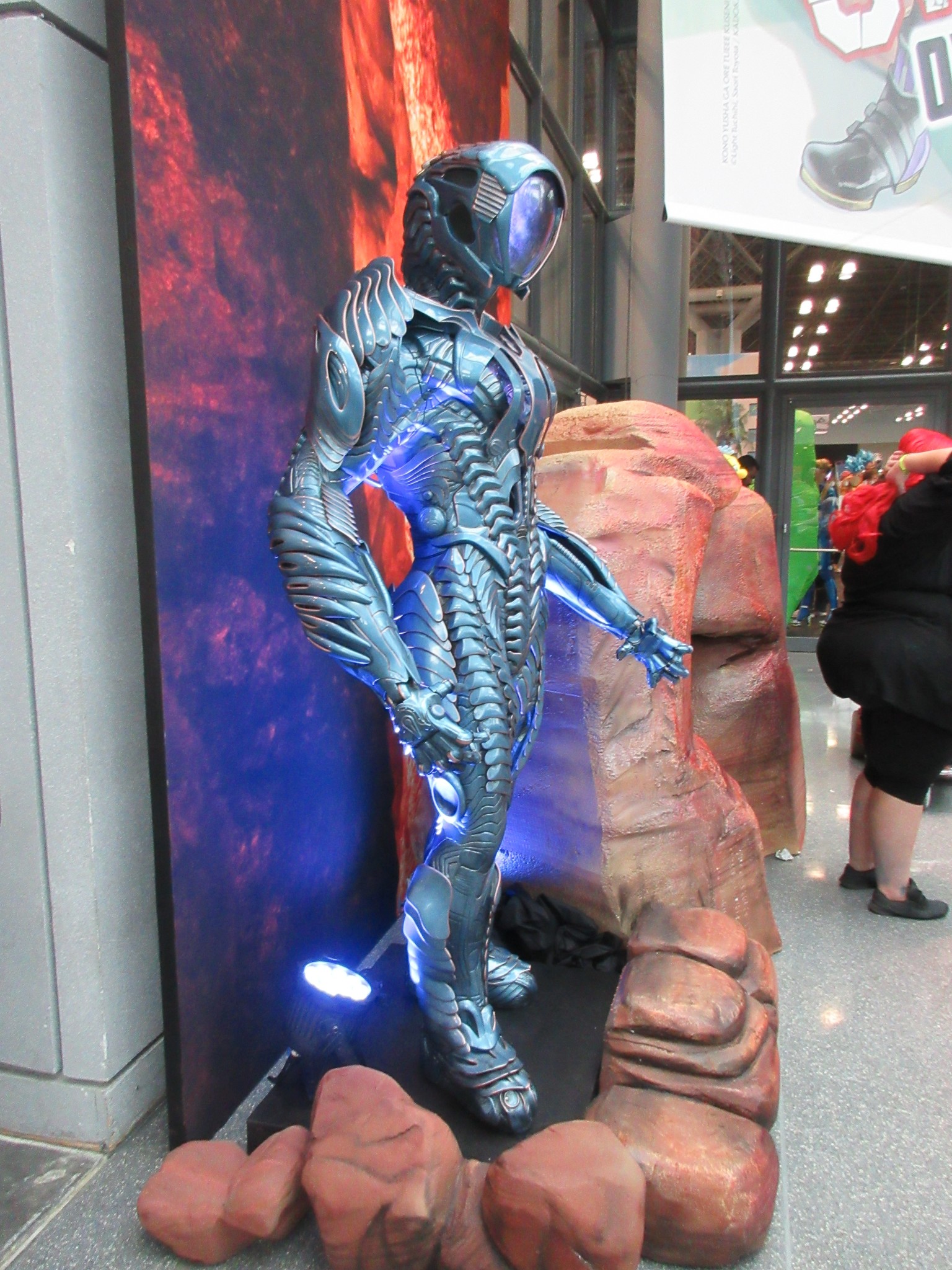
[788,451,886,626]
[277,142,704,1141]
[815,429,950,918]
[736,454,764,488]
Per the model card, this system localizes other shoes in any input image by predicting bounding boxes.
[788,607,838,628]
[868,878,950,921]
[839,864,877,890]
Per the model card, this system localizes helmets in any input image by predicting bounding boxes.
[407,141,567,302]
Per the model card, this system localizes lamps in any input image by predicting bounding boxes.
[270,945,378,1110]
